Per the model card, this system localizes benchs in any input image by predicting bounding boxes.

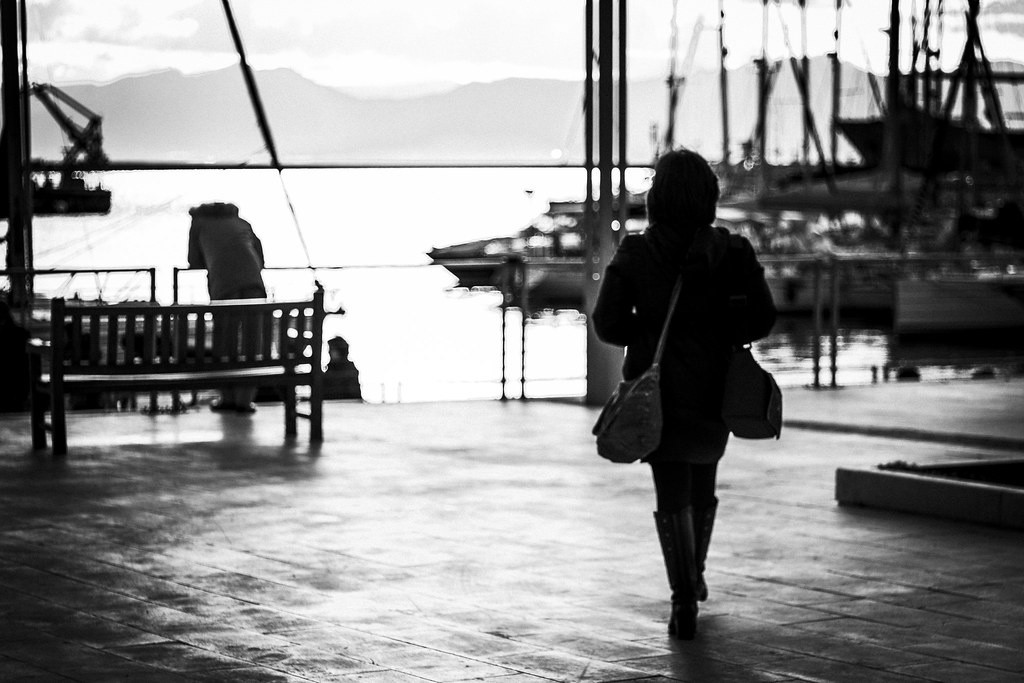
[24,292,326,457]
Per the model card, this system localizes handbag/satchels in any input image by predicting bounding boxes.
[723,348,783,439]
[591,366,664,464]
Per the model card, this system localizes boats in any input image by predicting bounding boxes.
[425,69,1024,353]
[30,155,111,218]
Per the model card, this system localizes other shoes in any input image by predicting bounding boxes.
[208,397,256,412]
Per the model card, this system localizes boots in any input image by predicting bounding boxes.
[696,495,718,601]
[655,510,697,638]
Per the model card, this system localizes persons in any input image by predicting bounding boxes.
[592,150,776,635]
[322,336,361,400]
[188,203,267,413]
